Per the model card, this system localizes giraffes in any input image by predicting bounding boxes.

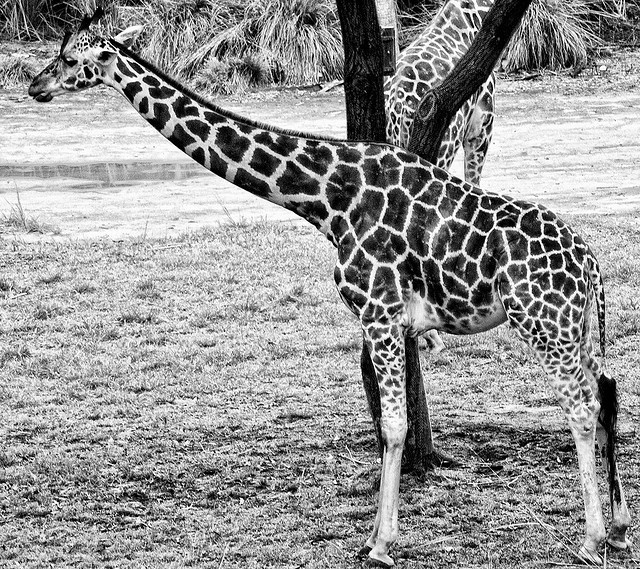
[382,0,497,357]
[28,5,635,568]
